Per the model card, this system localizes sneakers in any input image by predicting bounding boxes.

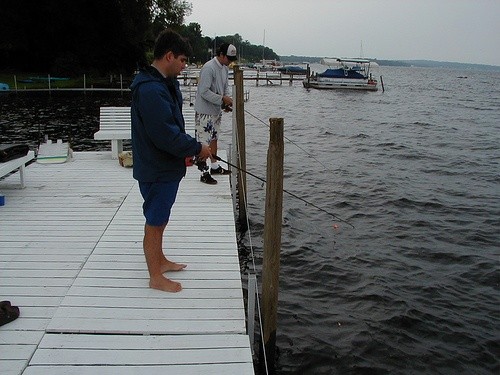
[210,165,232,175]
[200,172,217,184]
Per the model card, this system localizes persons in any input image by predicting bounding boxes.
[195,43,238,185]
[131,30,214,293]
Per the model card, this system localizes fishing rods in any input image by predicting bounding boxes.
[189,152,356,230]
[220,99,338,178]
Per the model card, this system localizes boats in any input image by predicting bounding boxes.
[320,58,380,68]
[277,66,307,75]
[301,63,378,91]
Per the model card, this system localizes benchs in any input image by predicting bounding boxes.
[94,107,195,160]
[0,151,35,189]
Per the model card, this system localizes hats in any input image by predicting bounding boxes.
[220,43,238,61]
